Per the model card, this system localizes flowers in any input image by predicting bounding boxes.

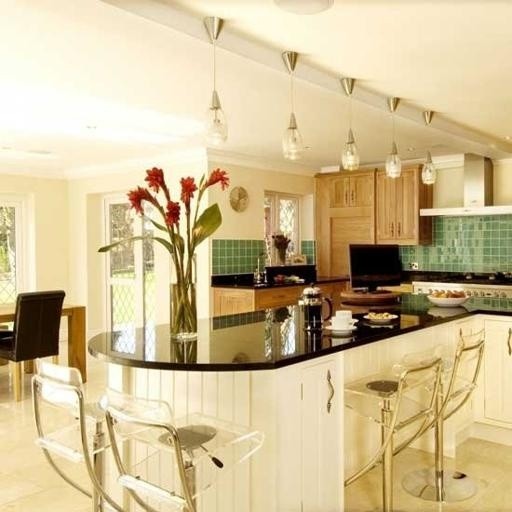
[96,165,230,334]
[272,234,291,261]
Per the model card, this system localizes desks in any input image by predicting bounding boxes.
[0,299,88,384]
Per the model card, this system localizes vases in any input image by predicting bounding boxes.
[277,249,286,265]
[168,254,198,343]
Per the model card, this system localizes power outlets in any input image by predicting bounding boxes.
[411,262,418,270]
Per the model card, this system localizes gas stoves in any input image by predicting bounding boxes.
[461,271,512,284]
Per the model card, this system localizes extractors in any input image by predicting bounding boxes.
[420,153,511,217]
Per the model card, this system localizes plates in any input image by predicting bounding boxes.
[329,334,359,341]
[324,316,360,334]
[363,315,400,322]
[362,322,400,328]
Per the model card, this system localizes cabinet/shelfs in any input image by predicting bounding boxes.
[214,281,347,316]
[486,320,512,425]
[314,162,433,278]
[302,360,339,512]
[338,290,401,314]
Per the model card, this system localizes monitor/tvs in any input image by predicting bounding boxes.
[348,243,401,292]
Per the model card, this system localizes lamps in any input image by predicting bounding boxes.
[384,96,403,180]
[280,51,304,163]
[421,111,436,185]
[202,16,229,147]
[341,77,360,173]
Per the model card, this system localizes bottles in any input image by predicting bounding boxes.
[254,269,269,284]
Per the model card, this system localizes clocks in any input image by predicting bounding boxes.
[229,186,249,212]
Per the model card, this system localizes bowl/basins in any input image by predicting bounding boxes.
[427,307,470,317]
[428,294,470,306]
[340,303,403,309]
[340,289,404,302]
[274,277,296,283]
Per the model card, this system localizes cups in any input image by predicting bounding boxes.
[304,331,333,354]
[333,308,354,327]
[332,339,356,349]
[298,283,333,330]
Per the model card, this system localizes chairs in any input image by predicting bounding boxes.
[344,326,485,512]
[0,290,65,402]
[31,356,265,512]
